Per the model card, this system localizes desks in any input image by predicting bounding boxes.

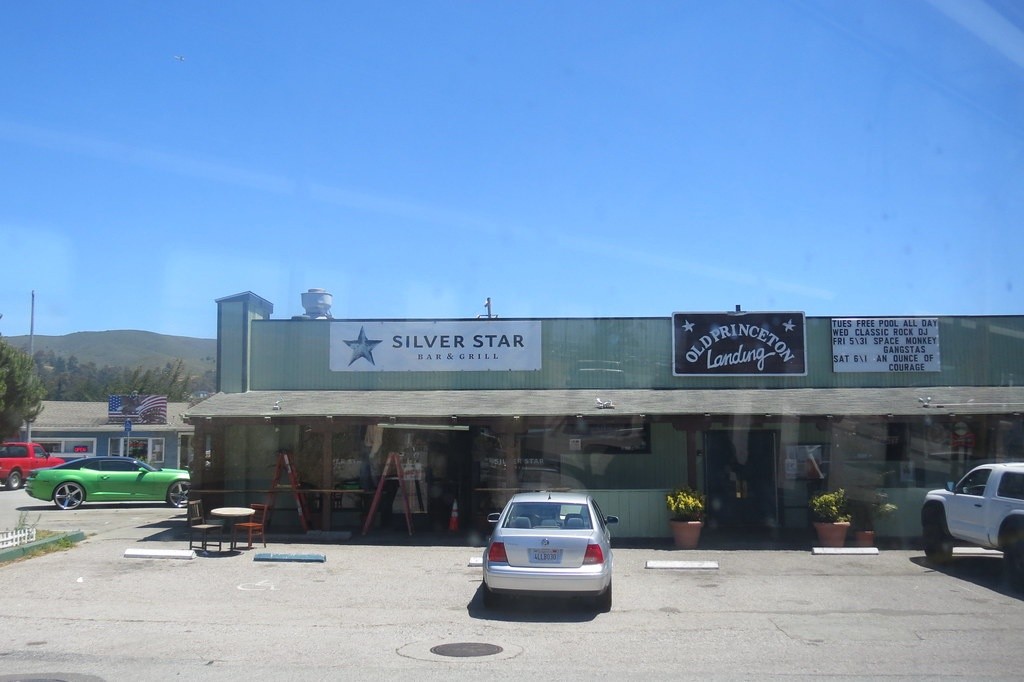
[210,507,255,556]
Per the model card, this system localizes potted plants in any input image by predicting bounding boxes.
[665,485,709,550]
[853,505,885,547]
[808,488,852,547]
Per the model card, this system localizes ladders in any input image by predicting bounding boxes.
[361,452,415,536]
[266,447,312,534]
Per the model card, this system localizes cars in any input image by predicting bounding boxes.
[25,455,189,510]
[482,491,617,613]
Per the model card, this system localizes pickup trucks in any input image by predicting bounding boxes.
[922,459,1024,583]
[0,441,66,489]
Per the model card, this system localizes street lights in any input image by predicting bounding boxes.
[484,297,492,319]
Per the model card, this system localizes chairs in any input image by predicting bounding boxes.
[188,499,223,552]
[233,503,267,550]
[513,513,584,528]
